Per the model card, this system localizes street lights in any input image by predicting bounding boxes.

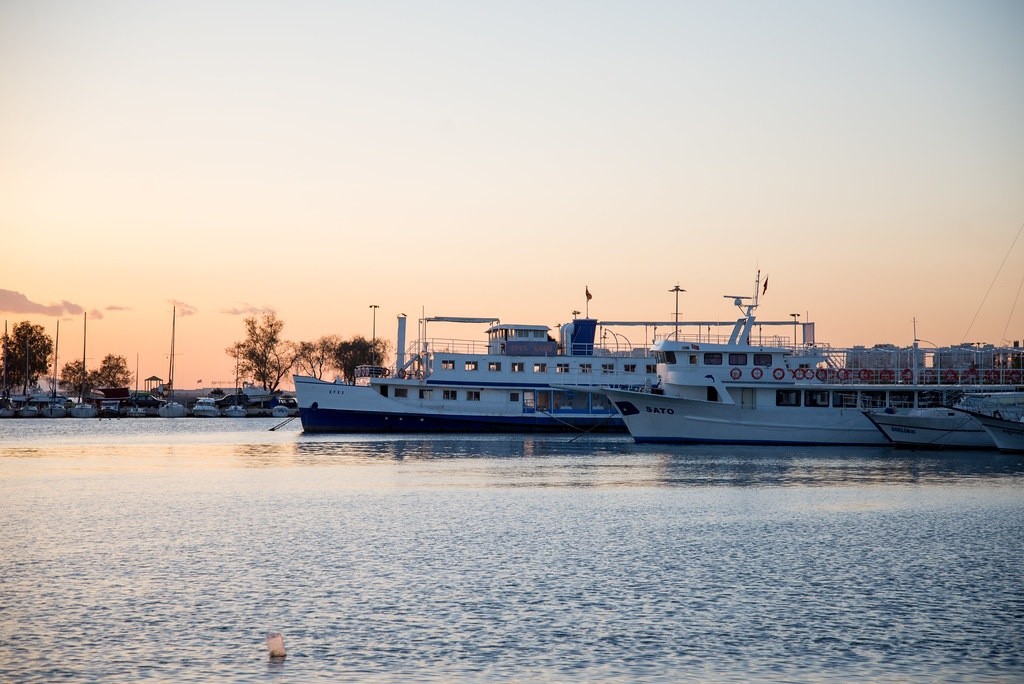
[369,304,380,376]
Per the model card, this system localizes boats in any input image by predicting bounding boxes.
[600,270,1024,451]
[98,399,120,418]
[292,317,660,435]
[225,406,248,416]
[272,406,289,417]
[193,399,220,417]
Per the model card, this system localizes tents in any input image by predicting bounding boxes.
[144,375,163,382]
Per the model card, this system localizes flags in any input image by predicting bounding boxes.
[197,379,203,383]
[762,276,768,296]
[585,287,592,303]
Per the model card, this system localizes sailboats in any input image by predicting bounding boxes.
[0,319,18,418]
[43,320,65,418]
[20,339,42,417]
[70,312,97,418]
[128,305,189,417]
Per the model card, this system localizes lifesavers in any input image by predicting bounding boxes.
[816,368,827,381]
[804,368,814,380]
[941,368,1021,384]
[787,368,795,379]
[729,368,742,381]
[795,368,805,380]
[772,367,785,381]
[397,368,408,379]
[837,368,914,381]
[381,368,391,377]
[750,367,763,380]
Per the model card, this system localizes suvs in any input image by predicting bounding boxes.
[212,394,250,408]
